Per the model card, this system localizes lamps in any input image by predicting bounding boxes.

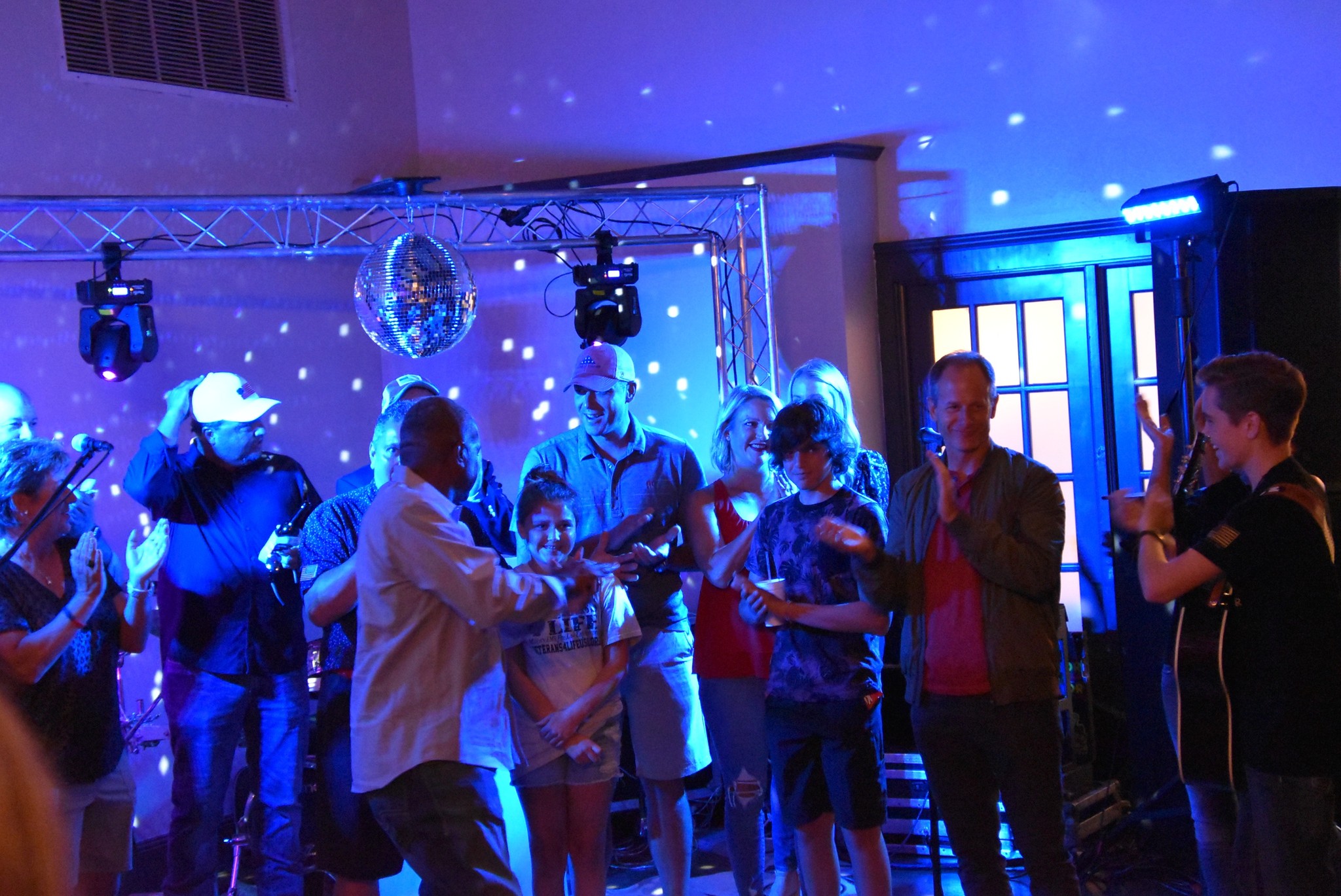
[352,203,477,360]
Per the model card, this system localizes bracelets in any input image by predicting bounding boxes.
[62,606,87,629]
[1136,528,1169,548]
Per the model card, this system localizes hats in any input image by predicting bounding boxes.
[192,371,282,423]
[561,342,636,393]
[380,372,441,413]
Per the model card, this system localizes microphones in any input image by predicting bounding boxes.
[71,434,114,452]
[917,427,942,444]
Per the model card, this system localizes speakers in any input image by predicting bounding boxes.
[1150,185,1341,495]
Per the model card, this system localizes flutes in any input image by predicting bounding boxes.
[1173,430,1205,498]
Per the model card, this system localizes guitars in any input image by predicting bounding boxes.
[1172,603,1244,793]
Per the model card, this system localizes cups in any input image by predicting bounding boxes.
[755,578,787,626]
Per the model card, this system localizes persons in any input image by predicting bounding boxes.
[1105,353,1340,895]
[0,340,893,896]
[815,351,1069,896]
